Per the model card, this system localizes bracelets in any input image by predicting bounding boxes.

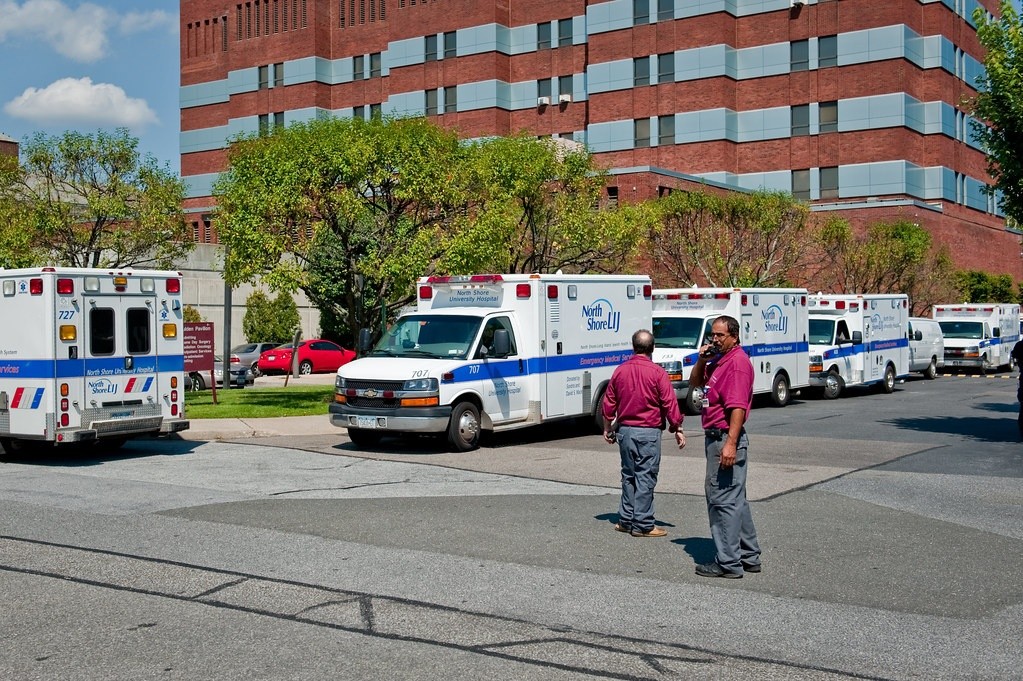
[676,430,683,434]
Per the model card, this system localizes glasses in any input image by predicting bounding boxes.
[708,333,732,342]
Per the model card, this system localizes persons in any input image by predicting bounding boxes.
[602,329,686,537]
[688,316,762,578]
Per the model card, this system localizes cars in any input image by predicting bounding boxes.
[258,338,358,374]
[228,343,284,378]
[182,354,255,392]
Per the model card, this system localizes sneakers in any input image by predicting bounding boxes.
[746,565,761,571]
[632,525,667,537]
[615,523,631,532]
[695,558,743,578]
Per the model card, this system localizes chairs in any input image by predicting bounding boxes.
[418,324,443,344]
[656,325,678,337]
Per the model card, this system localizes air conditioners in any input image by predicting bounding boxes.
[792,0,807,6]
[559,94,571,103]
[538,96,549,105]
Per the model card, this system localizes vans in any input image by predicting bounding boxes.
[908,317,946,380]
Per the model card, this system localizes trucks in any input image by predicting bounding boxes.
[648,287,813,416]
[329,273,656,453]
[1019,312,1023,347]
[0,266,190,462]
[804,291,912,398]
[932,303,1021,375]
